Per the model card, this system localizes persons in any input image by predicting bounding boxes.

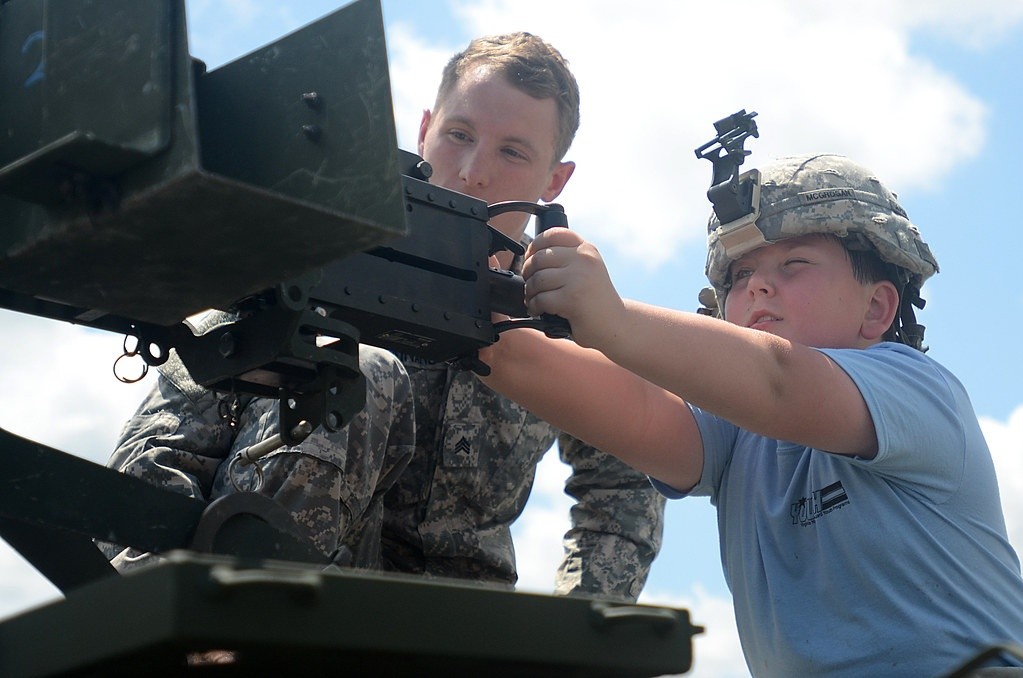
[475,107,1021,676]
[87,30,669,604]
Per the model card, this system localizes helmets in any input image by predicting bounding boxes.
[704,153,940,321]
